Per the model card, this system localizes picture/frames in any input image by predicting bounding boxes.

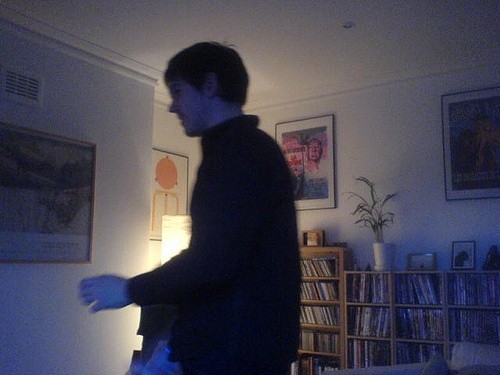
[451,240,476,271]
[406,252,436,271]
[274,113,337,211]
[440,85,500,202]
[302,229,326,247]
[0,120,97,265]
[149,147,190,241]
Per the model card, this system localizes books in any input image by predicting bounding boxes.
[294,257,500,374]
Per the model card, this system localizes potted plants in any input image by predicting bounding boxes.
[341,174,399,271]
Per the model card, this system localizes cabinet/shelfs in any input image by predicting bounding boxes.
[292,246,500,375]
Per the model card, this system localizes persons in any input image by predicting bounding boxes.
[77,40,303,374]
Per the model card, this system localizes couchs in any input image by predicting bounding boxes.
[323,341,500,375]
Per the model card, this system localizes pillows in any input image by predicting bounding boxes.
[419,357,500,375]
[450,341,500,366]
